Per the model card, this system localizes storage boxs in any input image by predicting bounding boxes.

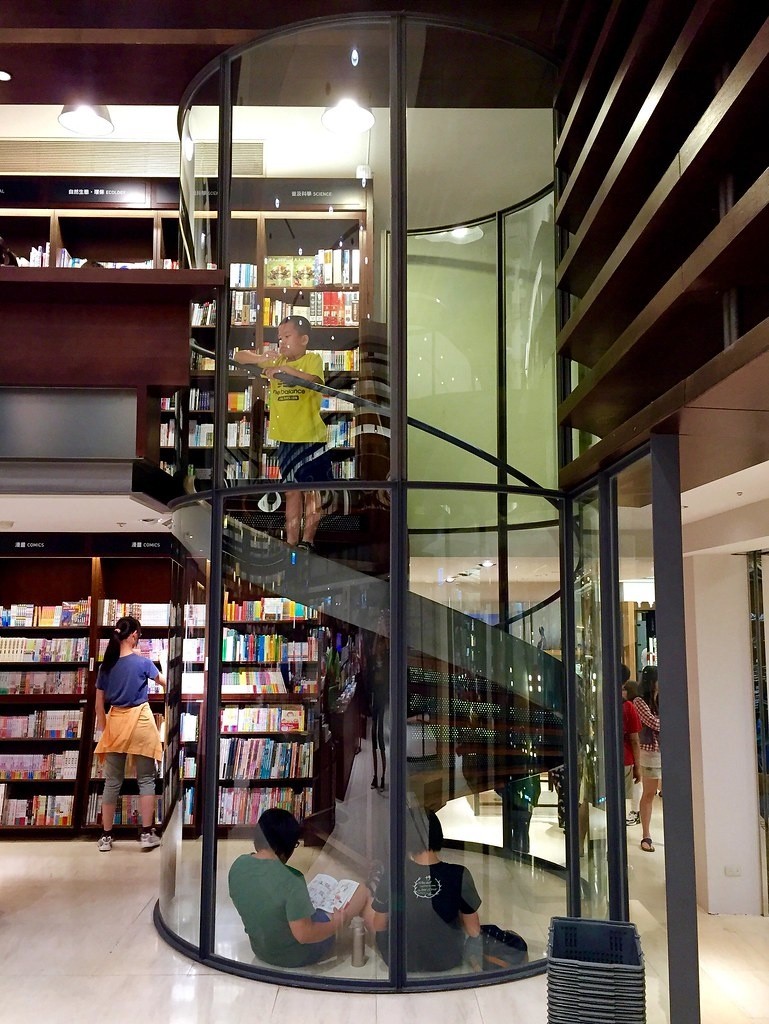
[546,916,646,1024]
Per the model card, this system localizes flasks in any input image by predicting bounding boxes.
[349,916,366,968]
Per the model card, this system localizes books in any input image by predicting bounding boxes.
[0,597,358,828]
[305,873,358,916]
[9,242,363,515]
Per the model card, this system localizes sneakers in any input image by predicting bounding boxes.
[98,836,112,851]
[139,828,162,850]
[626,811,640,827]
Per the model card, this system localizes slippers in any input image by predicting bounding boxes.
[641,837,656,853]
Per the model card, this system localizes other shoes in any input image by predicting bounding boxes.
[298,541,314,554]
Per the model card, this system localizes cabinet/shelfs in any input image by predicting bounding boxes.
[0,208,368,516]
[553,0,769,509]
[0,551,338,846]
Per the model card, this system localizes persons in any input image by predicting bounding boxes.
[578,717,606,907]
[506,773,540,856]
[233,314,332,556]
[535,626,548,651]
[359,805,482,975]
[369,628,392,794]
[95,615,168,854]
[620,677,643,827]
[616,663,643,800]
[549,763,567,836]
[226,807,389,967]
[633,665,663,853]
